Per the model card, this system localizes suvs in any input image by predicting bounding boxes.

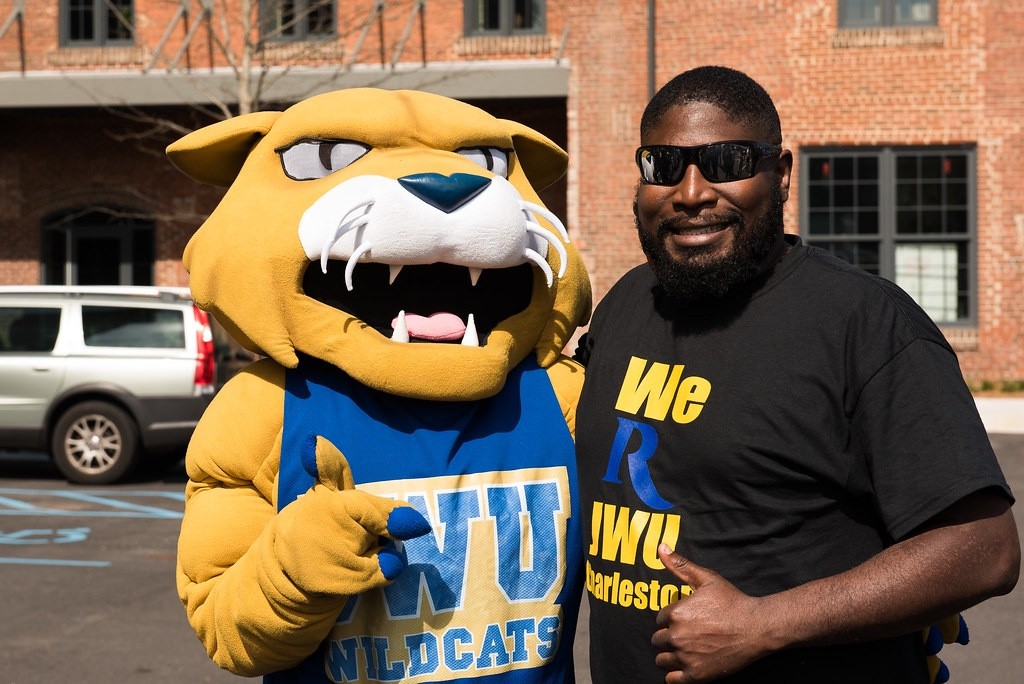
[0,285,258,486]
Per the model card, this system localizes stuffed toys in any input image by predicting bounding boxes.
[166,88,592,684]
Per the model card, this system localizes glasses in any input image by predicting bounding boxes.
[636,141,782,187]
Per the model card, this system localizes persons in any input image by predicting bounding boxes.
[572,66,1021,684]
